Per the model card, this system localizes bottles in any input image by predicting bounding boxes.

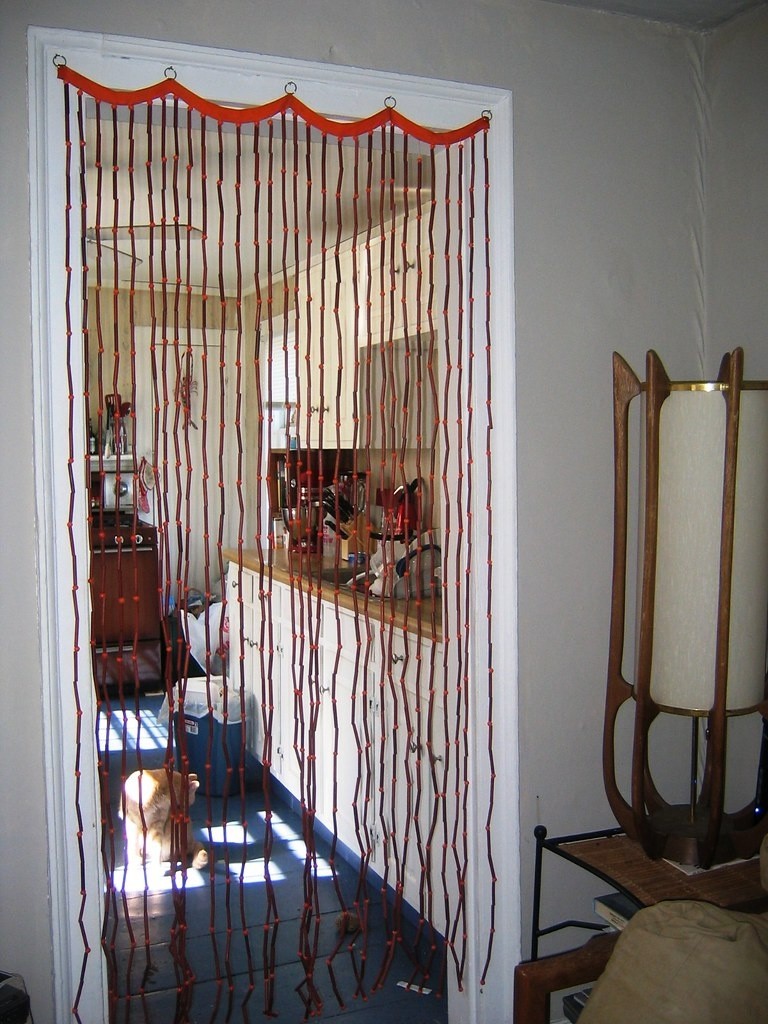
[322,513,336,557]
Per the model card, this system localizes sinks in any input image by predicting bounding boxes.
[304,565,378,600]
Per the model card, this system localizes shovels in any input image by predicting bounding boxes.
[105,394,124,455]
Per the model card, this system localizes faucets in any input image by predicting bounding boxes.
[347,569,374,587]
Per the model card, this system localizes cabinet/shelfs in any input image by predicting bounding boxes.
[89,518,165,693]
[222,549,446,938]
[299,213,437,448]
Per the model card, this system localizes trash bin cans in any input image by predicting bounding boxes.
[172,676,252,797]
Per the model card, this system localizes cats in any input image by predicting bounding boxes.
[118,768,209,872]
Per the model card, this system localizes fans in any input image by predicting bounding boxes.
[84,224,202,267]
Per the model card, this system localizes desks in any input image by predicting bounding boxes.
[529,822,768,962]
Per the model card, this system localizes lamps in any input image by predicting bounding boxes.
[602,348,768,870]
